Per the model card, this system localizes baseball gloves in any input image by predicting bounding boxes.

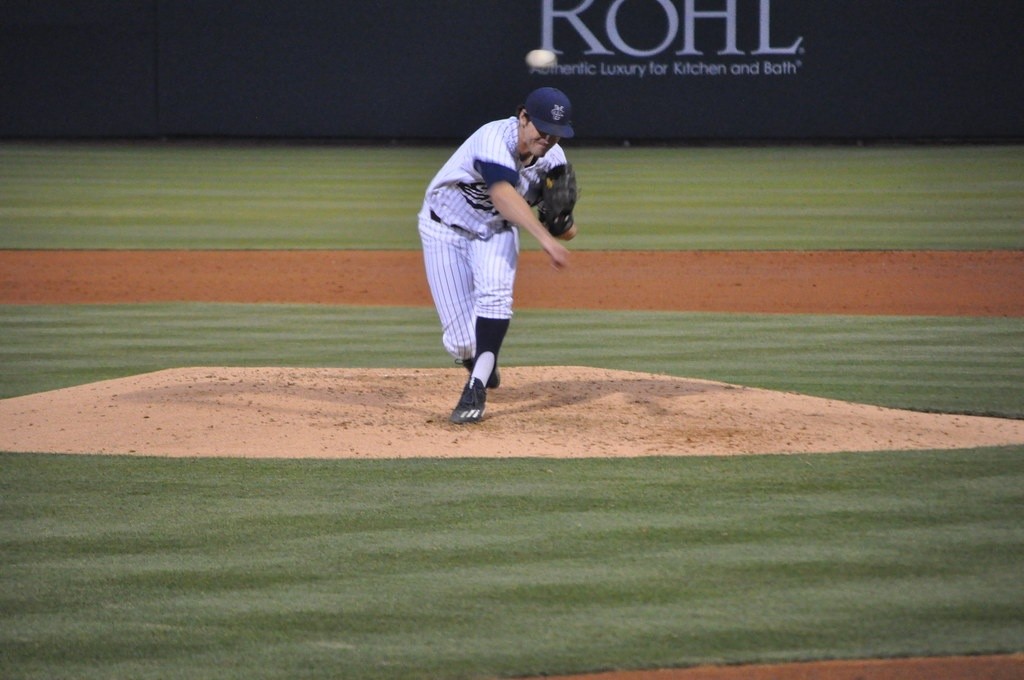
[535,164,577,236]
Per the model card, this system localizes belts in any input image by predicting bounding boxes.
[430,210,470,234]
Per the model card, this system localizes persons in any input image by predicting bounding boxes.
[418,86,579,425]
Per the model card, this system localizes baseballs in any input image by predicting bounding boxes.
[528,48,555,68]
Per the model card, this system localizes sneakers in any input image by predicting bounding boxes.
[455,357,500,389]
[450,384,487,425]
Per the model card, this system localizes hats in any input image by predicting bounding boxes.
[524,88,575,138]
